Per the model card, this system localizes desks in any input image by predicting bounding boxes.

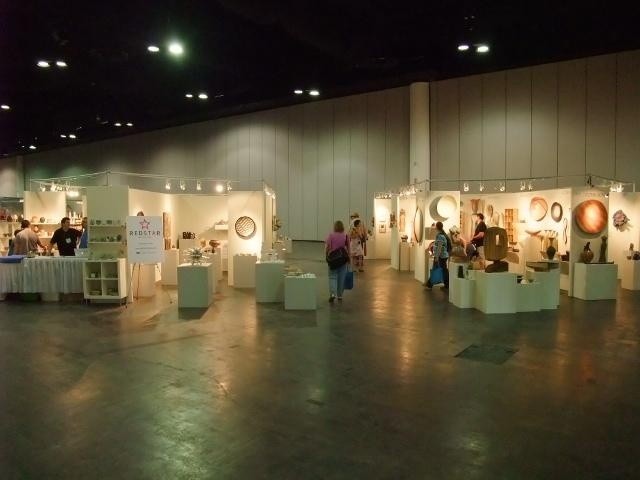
[0,257,88,302]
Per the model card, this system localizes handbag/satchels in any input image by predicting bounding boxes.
[344,272,353,288]
[447,239,452,251]
[430,268,443,285]
[326,248,349,270]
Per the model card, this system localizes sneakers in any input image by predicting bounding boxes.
[329,295,341,302]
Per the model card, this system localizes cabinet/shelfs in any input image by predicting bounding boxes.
[0,221,81,255]
[83,224,130,304]
[504,208,518,243]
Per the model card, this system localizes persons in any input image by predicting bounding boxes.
[471,213,486,270]
[14,219,47,255]
[422,221,452,290]
[79,217,87,248]
[349,220,365,272]
[325,220,351,301]
[350,212,368,256]
[47,217,82,256]
[8,230,22,256]
